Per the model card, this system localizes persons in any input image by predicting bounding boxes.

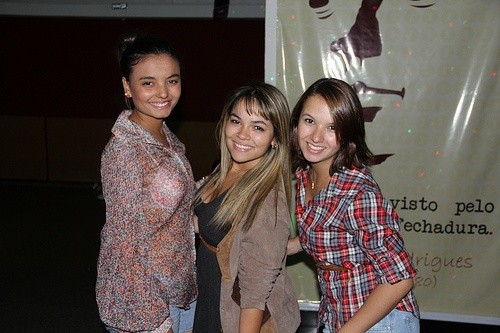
[190,83,301,333]
[100,36,207,333]
[285,77,421,333]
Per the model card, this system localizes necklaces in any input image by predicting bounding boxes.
[308,171,331,188]
